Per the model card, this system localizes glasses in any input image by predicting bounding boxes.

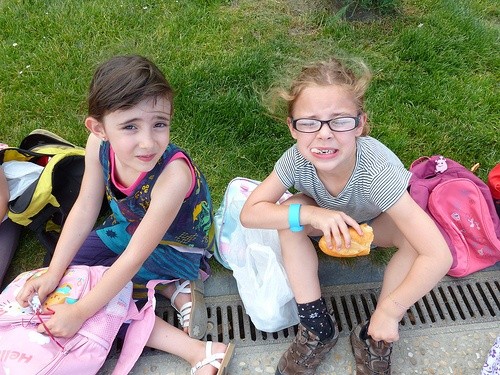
[290,113,361,133]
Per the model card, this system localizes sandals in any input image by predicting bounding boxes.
[190,340,236,375]
[171,278,208,340]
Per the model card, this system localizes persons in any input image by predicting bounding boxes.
[239,56,454,375]
[15,54,236,375]
[0,165,21,285]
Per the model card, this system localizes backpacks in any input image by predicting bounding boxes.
[0,129,109,255]
[214,177,294,270]
[407,154,500,278]
[0,264,156,375]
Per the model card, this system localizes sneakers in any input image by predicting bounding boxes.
[274,308,340,375]
[350,309,394,375]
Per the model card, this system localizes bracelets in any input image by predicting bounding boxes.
[288,203,304,232]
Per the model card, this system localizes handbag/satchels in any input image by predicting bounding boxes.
[229,243,299,333]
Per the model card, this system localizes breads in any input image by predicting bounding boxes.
[318,223,375,257]
